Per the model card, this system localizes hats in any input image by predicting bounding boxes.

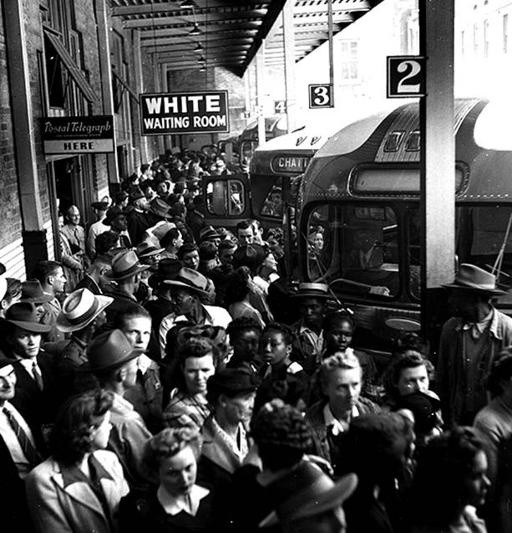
[295,282,336,297]
[440,264,509,298]
[0,352,24,369]
[91,160,268,296]
[76,329,145,373]
[205,369,258,402]
[55,287,114,334]
[0,302,53,333]
[255,459,359,531]
[19,278,52,301]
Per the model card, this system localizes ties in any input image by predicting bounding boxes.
[32,364,44,391]
[2,407,43,468]
